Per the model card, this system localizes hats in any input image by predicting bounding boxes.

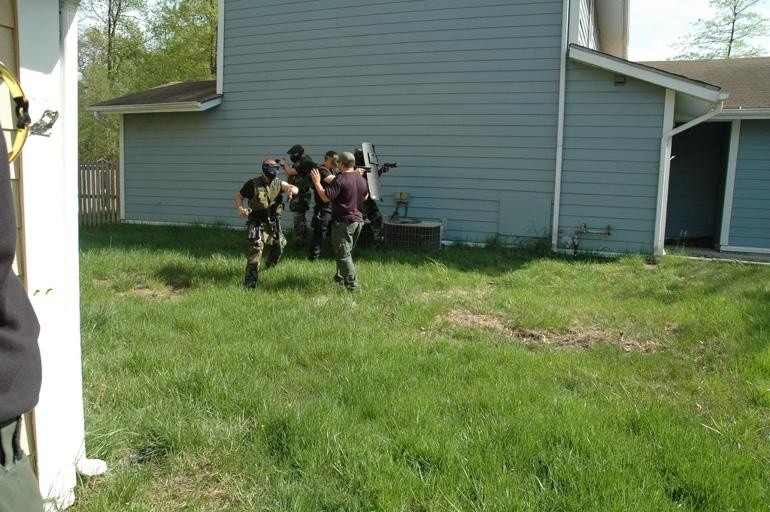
[333,151,356,168]
[286,145,304,154]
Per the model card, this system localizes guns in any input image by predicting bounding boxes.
[354,166,371,173]
[384,163,397,168]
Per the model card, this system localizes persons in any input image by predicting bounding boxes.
[308,150,366,260]
[233,159,300,288]
[354,145,390,253]
[278,144,313,246]
[309,152,369,291]
